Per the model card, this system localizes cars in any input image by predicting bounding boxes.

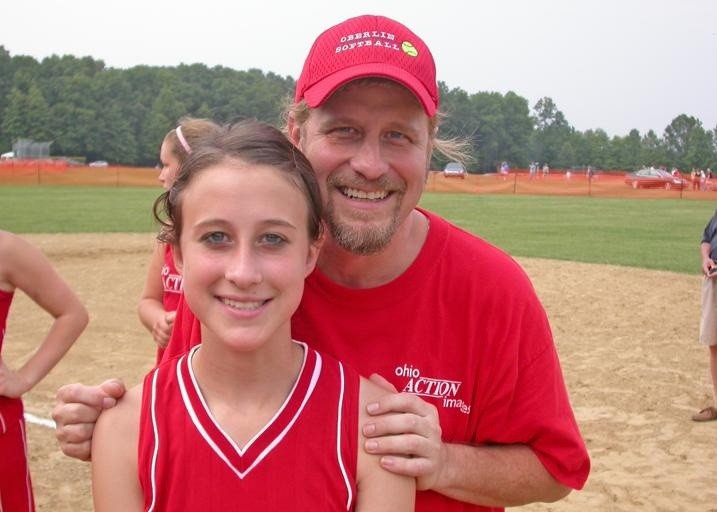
[0,150,14,160]
[66,158,84,167]
[622,168,689,191]
[88,160,108,168]
[443,160,465,181]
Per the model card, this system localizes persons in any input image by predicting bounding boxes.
[691,208,716,422]
[135,116,235,375]
[496,153,714,197]
[52,15,591,512]
[92,116,423,512]
[0,224,89,512]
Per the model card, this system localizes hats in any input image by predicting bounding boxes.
[295,16,439,117]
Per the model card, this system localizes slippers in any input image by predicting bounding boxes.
[693,407,717,421]
